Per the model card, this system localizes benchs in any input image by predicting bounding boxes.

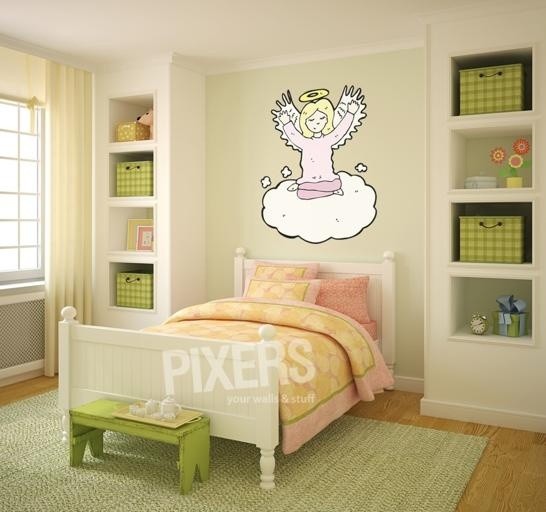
[69,398,210,495]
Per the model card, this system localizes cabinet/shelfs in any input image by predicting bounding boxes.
[417,0,546,435]
[90,52,207,332]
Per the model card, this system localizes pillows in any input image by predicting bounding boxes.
[244,261,372,324]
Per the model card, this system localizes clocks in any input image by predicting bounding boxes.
[470,312,487,335]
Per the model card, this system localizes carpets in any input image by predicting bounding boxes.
[0,386,490,512]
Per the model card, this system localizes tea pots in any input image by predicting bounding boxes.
[145,399,161,415]
[161,396,181,419]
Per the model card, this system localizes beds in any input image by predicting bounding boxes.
[57,247,398,491]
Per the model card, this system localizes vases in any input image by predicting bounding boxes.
[507,177,522,188]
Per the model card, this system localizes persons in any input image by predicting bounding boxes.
[136,108,153,138]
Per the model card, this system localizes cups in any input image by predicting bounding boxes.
[129,405,147,418]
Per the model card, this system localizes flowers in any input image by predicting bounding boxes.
[489,137,531,177]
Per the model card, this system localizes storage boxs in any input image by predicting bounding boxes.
[459,63,525,115]
[457,214,524,264]
[115,120,150,141]
[114,271,152,309]
[114,160,152,197]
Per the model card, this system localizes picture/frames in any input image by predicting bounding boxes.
[135,225,153,251]
[127,219,154,251]
